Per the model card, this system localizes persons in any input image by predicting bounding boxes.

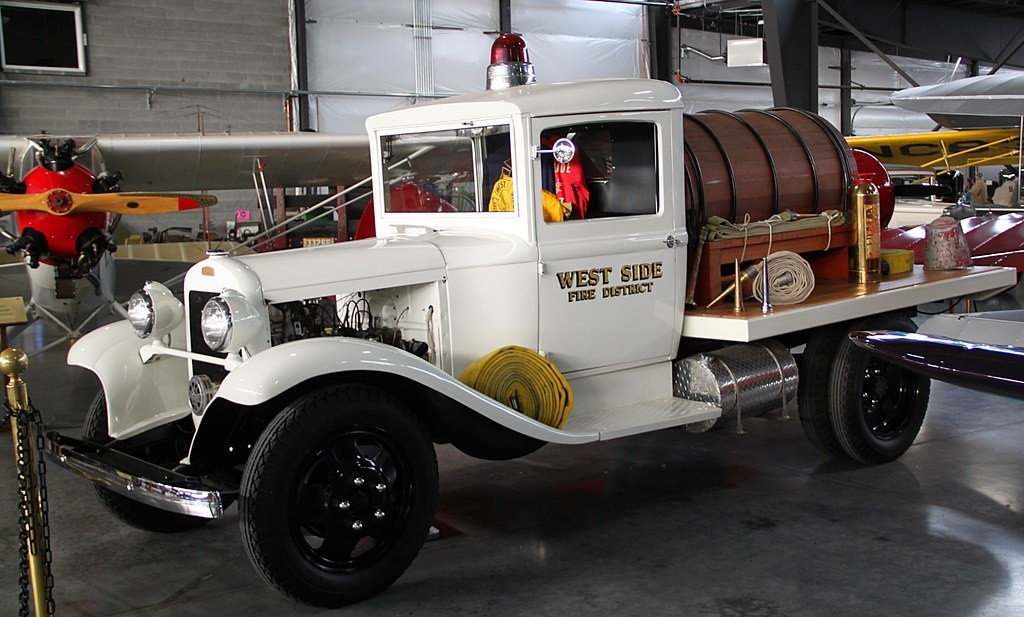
[962,173,986,204]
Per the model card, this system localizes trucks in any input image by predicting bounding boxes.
[41,30,1019,609]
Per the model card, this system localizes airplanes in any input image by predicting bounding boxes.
[0,130,473,300]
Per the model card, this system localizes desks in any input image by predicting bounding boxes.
[701,225,849,307]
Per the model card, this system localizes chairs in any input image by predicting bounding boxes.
[575,137,606,219]
